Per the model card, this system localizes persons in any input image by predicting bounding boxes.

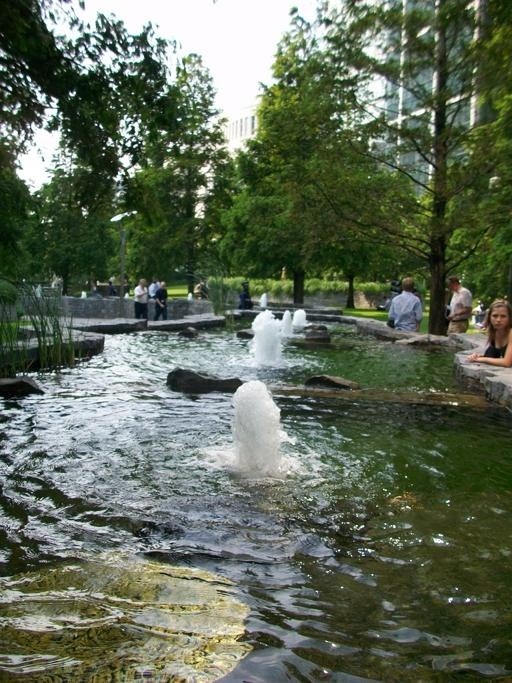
[377,276,512,368]
[107,278,253,321]
